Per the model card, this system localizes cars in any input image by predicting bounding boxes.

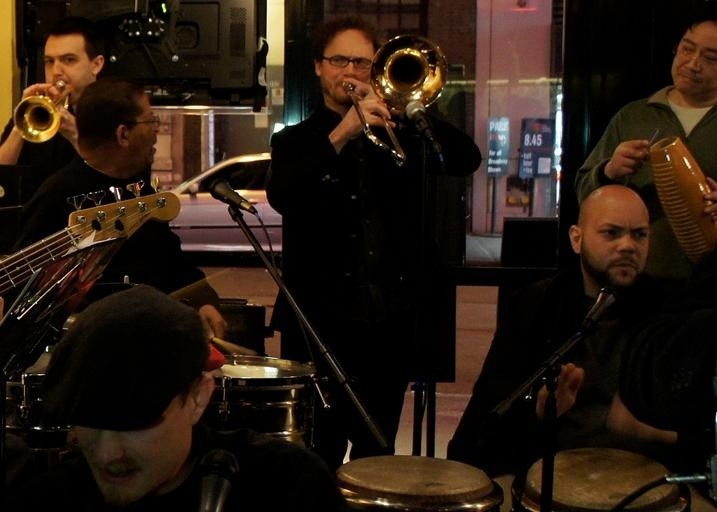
[169,152,284,253]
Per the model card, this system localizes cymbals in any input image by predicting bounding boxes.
[219,355,317,380]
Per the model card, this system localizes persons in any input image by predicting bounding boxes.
[266,18,483,460]
[15,283,353,510]
[13,78,230,348]
[0,15,163,198]
[565,12,715,291]
[471,184,709,470]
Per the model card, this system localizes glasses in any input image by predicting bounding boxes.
[137,115,160,129]
[322,55,371,70]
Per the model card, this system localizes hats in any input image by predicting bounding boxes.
[27,284,209,432]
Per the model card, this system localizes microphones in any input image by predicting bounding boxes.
[197,449,240,512]
[209,180,257,214]
[581,284,619,331]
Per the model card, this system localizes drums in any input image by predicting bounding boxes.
[510,447,690,510]
[3,347,74,436]
[192,384,318,453]
[337,456,504,511]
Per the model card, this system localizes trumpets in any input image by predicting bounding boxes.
[13,80,70,143]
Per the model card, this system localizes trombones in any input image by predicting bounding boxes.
[342,35,446,165]
